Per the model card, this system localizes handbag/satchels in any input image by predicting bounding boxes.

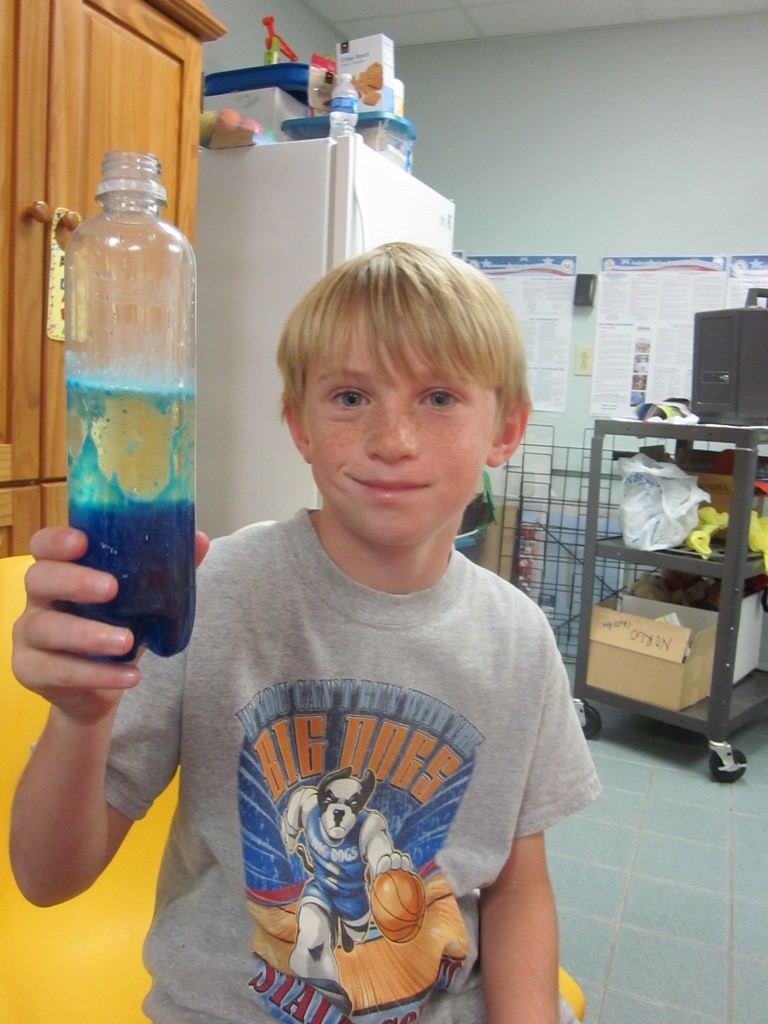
[619,454,710,551]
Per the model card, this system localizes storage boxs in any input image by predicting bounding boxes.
[279,111,418,177]
[335,33,395,114]
[206,86,315,141]
[693,469,735,539]
[616,586,766,684]
[587,596,717,711]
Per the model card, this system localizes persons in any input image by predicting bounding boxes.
[10,240,607,1024]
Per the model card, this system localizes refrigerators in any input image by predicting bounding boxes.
[195,134,459,543]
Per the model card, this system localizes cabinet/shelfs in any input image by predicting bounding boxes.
[0,0,227,552]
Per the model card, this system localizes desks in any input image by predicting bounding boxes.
[571,418,768,745]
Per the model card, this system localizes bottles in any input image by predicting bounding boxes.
[329,71,359,141]
[64,149,198,663]
